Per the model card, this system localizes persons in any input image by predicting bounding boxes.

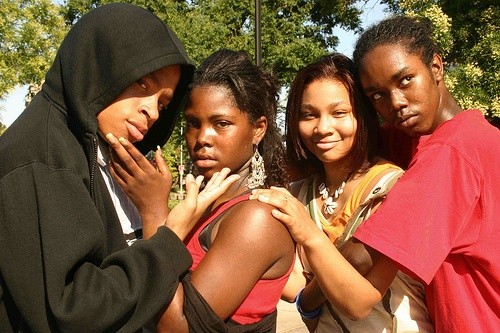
[0,5,241,333]
[105,49,295,333]
[247,15,500,333]
[248,52,435,333]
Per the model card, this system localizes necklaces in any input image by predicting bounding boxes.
[318,168,356,215]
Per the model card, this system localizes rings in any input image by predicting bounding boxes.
[284,196,288,204]
